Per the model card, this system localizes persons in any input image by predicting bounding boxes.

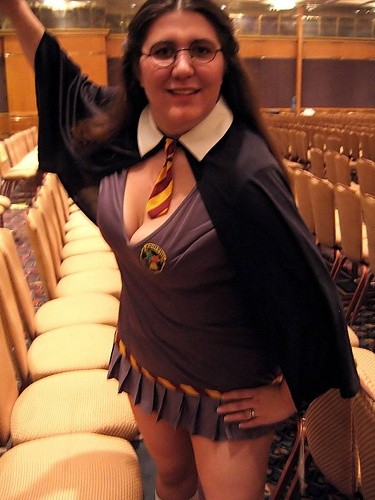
[0,0,362,500]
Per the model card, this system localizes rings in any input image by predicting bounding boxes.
[250,409,257,419]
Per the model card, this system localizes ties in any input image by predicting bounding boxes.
[146,139,177,219]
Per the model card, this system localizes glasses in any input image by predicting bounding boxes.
[141,41,222,67]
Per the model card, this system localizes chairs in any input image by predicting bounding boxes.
[0,126,147,500]
[262,106,375,500]
